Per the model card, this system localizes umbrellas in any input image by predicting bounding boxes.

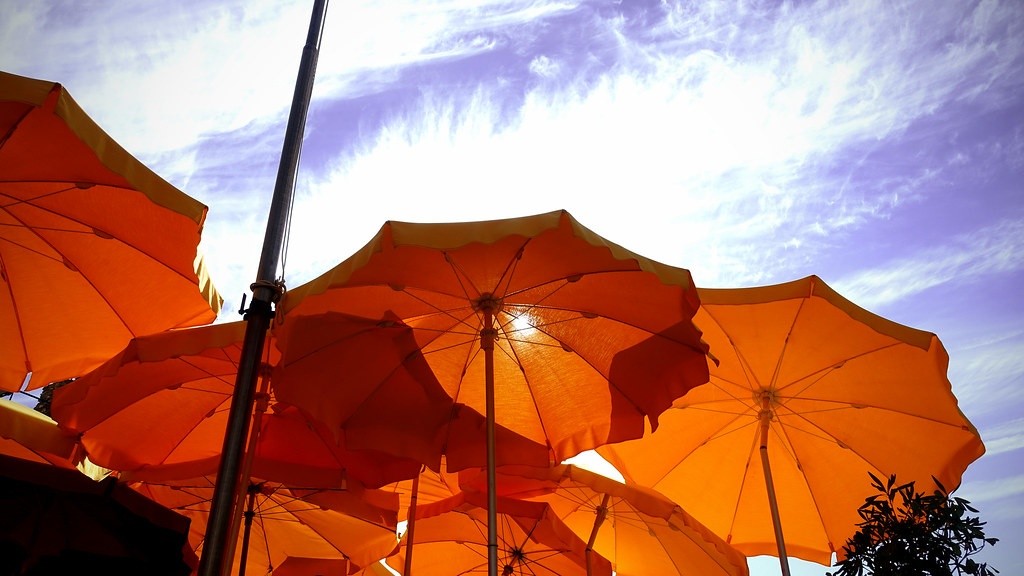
[596,275,986,575]
[52,311,453,575]
[278,209,710,576]
[1,402,747,576]
[1,72,221,394]
